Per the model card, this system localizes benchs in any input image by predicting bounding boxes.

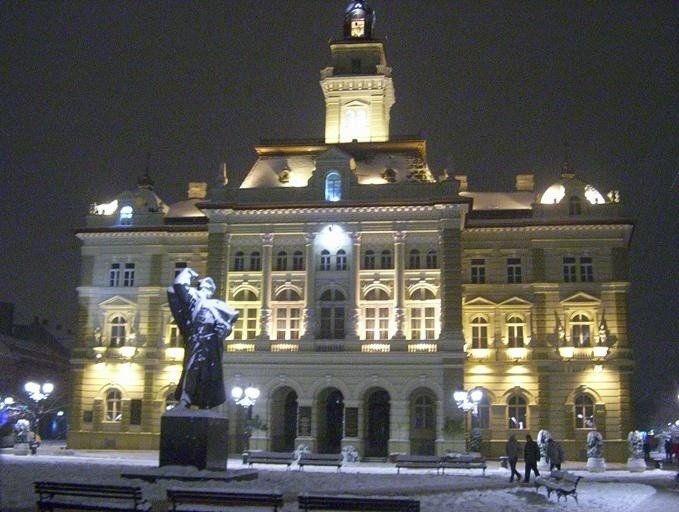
[297,495,420,511]
[33,481,151,511]
[247,451,343,474]
[534,470,583,505]
[395,454,486,475]
[166,488,283,512]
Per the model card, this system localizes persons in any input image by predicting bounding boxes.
[664,440,672,462]
[522,434,540,483]
[165,265,241,411]
[545,437,564,471]
[643,442,650,461]
[505,434,522,482]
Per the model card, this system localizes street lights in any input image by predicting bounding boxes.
[453,388,483,452]
[231,386,260,450]
[24,381,55,434]
